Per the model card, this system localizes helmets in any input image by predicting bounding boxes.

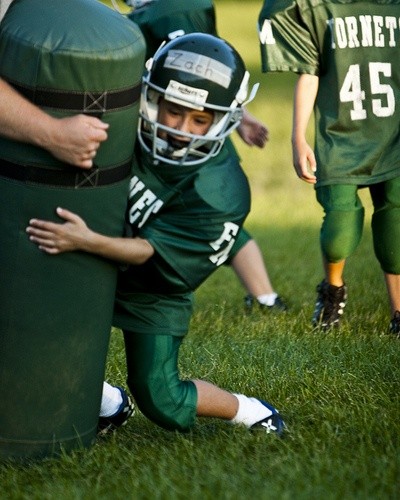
[147,33,248,114]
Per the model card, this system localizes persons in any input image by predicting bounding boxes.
[128,0,305,317]
[258,0,400,334]
[0,31,289,442]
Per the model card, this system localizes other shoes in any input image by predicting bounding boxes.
[243,292,302,317]
[243,396,284,455]
[387,310,400,337]
[312,277,348,330]
[98,385,135,438]
